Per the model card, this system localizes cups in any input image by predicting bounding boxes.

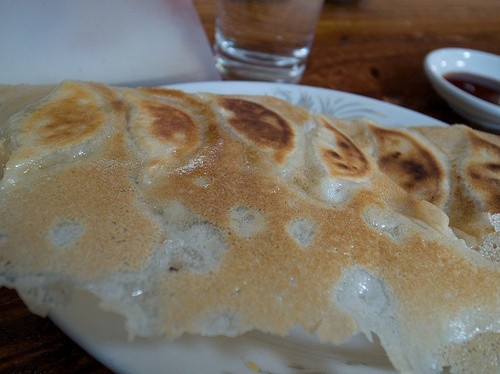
[213,0,324,83]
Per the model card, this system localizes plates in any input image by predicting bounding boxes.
[54,79,500,374]
[424,46,499,129]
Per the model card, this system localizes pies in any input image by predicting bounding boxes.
[0,79,500,373]
